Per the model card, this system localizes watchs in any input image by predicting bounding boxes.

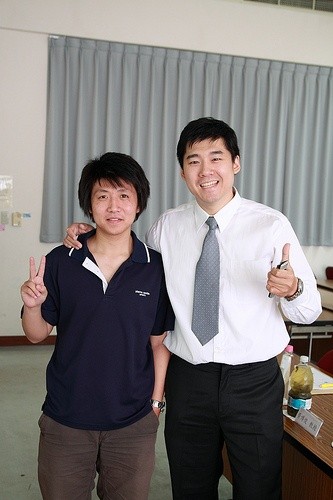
[284,278,304,301]
[149,399,166,415]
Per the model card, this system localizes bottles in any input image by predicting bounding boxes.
[280,345,294,407]
[287,356,313,418]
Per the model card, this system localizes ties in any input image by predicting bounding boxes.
[191,217,219,346]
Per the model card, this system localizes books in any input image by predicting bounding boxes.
[309,366,333,394]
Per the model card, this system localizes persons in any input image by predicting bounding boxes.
[20,151,175,500]
[64,118,322,500]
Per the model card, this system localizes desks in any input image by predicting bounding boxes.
[223,348,333,500]
